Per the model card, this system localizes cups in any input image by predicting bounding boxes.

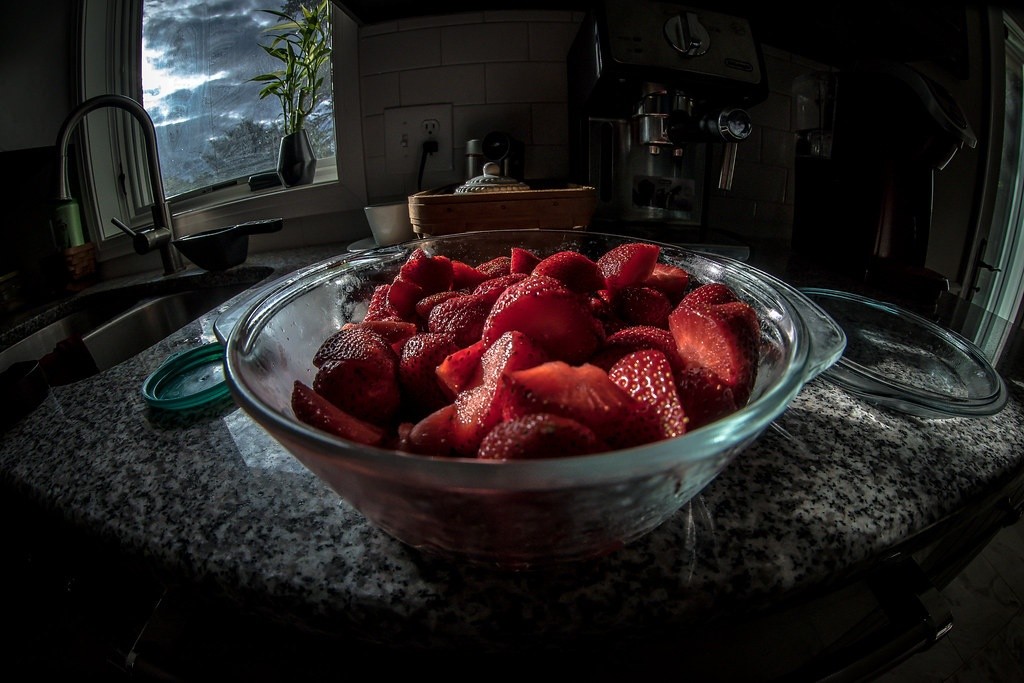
[364,200,408,247]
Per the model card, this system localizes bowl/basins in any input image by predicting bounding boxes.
[219,228,847,562]
[795,286,1010,420]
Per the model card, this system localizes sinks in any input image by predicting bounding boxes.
[27,264,276,388]
[1,275,168,385]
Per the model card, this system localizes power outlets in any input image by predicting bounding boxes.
[384,102,455,178]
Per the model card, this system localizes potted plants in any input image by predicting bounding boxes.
[241,0,334,189]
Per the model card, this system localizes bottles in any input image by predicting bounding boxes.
[46,200,84,246]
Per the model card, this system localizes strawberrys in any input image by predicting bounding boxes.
[290,244,762,564]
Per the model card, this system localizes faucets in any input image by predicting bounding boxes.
[53,93,187,276]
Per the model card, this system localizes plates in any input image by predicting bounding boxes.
[347,236,378,252]
